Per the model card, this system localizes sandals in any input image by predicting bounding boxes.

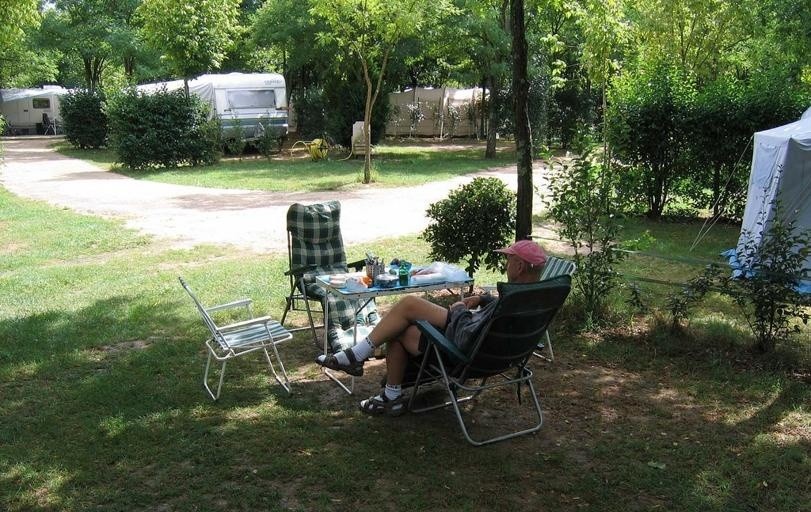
[315,348,363,376]
[358,391,407,417]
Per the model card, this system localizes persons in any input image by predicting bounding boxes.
[316,238,546,417]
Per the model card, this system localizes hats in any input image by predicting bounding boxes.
[492,241,547,265]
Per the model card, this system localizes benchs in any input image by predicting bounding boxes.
[11,125,36,135]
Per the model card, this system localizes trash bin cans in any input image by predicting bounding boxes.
[36,123,43,134]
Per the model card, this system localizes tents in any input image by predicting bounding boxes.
[719,104,811,294]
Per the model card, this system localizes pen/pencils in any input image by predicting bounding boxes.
[365,252,384,265]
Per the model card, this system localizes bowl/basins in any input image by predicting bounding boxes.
[374,274,399,287]
[328,276,347,289]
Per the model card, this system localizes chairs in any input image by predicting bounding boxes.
[280,199,379,353]
[382,251,575,448]
[176,275,296,402]
[41,112,56,134]
[351,119,376,159]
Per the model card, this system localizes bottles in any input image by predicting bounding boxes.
[399,262,409,286]
